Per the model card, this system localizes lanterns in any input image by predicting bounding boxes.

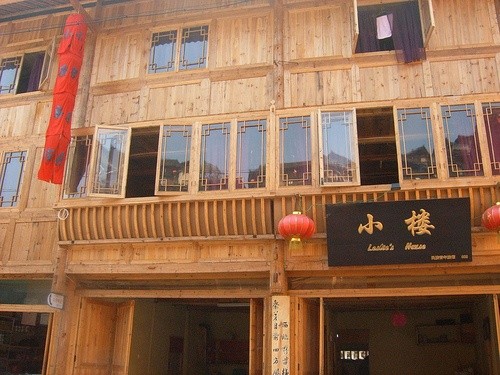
[277,210,316,251]
[482,201,500,231]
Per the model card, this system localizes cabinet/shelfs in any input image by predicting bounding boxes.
[415,321,478,345]
